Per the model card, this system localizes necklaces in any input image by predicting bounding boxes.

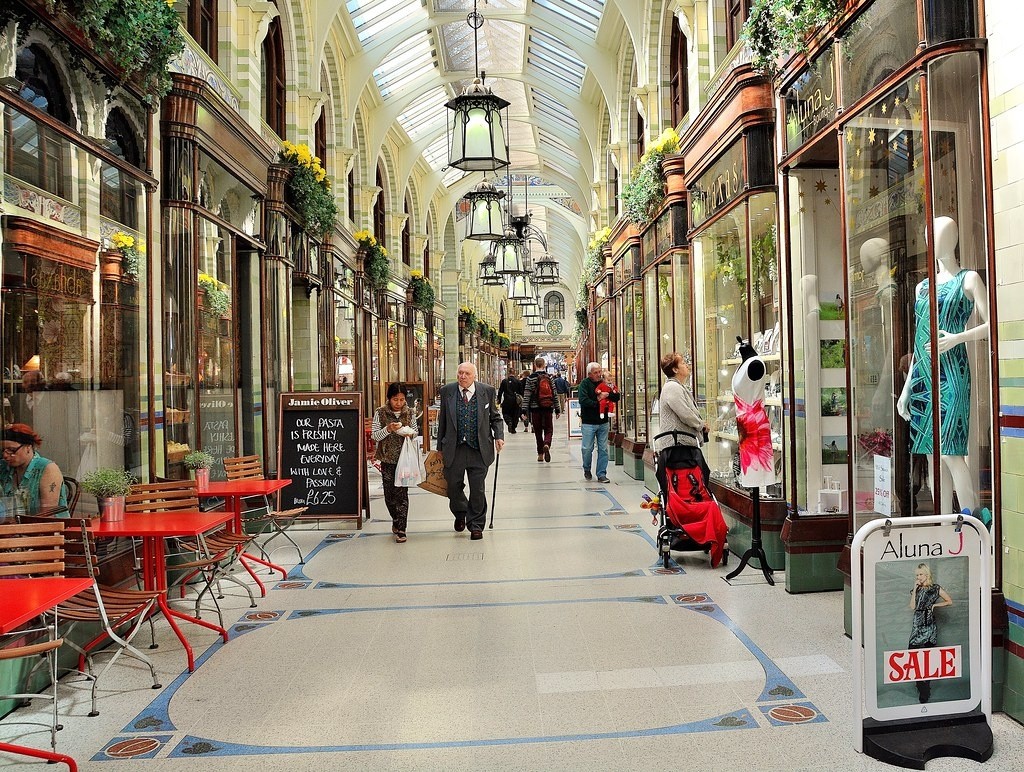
[754,327,779,348]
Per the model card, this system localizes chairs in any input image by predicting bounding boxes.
[0,454,308,765]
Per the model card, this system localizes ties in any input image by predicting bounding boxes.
[462,389,469,404]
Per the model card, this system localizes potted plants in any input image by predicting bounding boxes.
[184,451,216,488]
[80,465,139,522]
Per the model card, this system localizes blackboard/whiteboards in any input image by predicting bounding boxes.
[277,391,368,517]
[183,386,243,482]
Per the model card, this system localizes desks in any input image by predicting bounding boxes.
[0,506,68,523]
[0,578,96,772]
[180,479,292,598]
[65,512,235,674]
[818,488,848,512]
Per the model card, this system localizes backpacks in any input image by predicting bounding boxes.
[532,373,553,408]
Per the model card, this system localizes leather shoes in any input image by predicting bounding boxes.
[470,531,483,540]
[454,515,466,532]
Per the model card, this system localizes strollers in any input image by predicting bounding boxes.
[653,429,730,569]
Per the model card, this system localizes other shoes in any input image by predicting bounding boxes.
[543,445,551,462]
[538,455,544,461]
[524,428,528,432]
[512,429,516,434]
[584,471,592,480]
[598,476,610,483]
[508,422,512,433]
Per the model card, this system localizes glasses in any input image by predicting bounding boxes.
[0,445,24,454]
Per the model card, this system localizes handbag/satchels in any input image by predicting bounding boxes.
[418,448,448,497]
[394,436,427,488]
[515,394,524,407]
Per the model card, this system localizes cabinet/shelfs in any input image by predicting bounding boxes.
[714,355,780,452]
[164,366,194,480]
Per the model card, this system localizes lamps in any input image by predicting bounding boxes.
[444,1,561,333]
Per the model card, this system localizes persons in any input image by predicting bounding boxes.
[578,362,620,483]
[731,343,776,487]
[859,236,898,429]
[799,274,824,323]
[658,353,709,450]
[521,358,561,462]
[549,373,570,414]
[595,371,619,419]
[16,372,136,515]
[371,382,419,542]
[897,216,989,516]
[0,424,71,518]
[909,562,953,703]
[497,367,536,433]
[436,362,504,540]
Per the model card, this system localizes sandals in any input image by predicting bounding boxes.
[392,520,399,534]
[396,531,407,543]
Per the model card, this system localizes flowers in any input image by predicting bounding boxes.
[197,273,232,317]
[577,227,612,313]
[740,1,846,76]
[335,336,341,354]
[411,271,436,309]
[111,231,138,278]
[710,223,777,319]
[416,326,428,347]
[856,427,893,458]
[597,294,644,348]
[458,305,510,348]
[354,230,390,289]
[656,275,673,308]
[277,140,339,239]
[45,0,186,105]
[613,127,680,231]
